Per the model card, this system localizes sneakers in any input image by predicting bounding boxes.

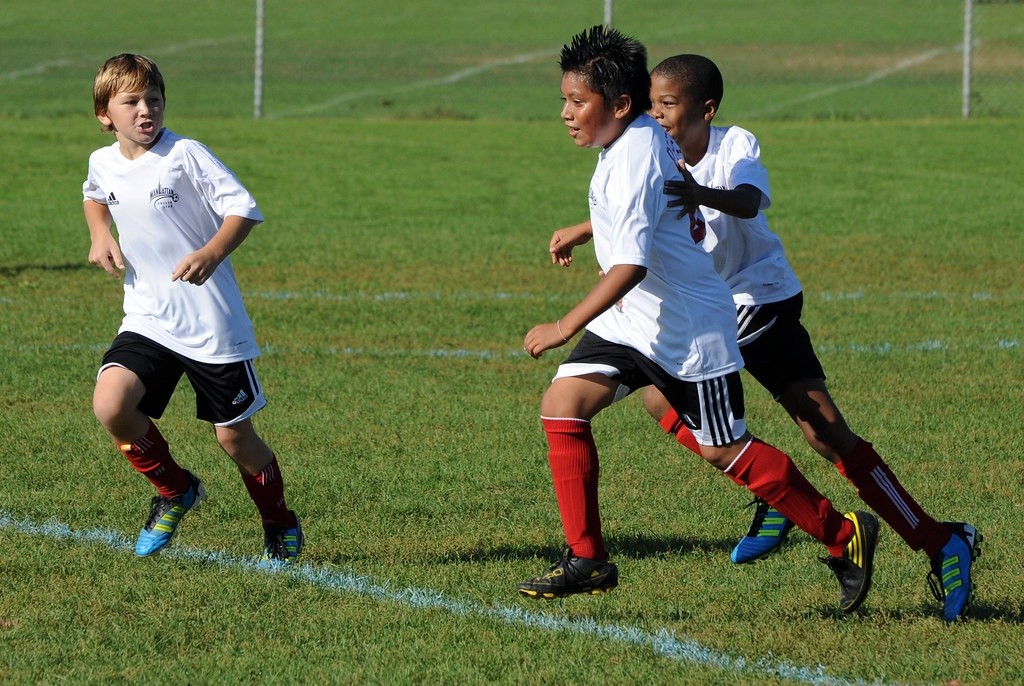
[818,510,881,614]
[926,522,983,623]
[730,497,795,564]
[134,469,205,557]
[518,547,617,599]
[259,510,304,574]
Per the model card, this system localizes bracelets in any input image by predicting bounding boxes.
[557,320,569,342]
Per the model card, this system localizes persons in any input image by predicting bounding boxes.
[518,23,883,615]
[546,53,985,623]
[76,49,307,578]
